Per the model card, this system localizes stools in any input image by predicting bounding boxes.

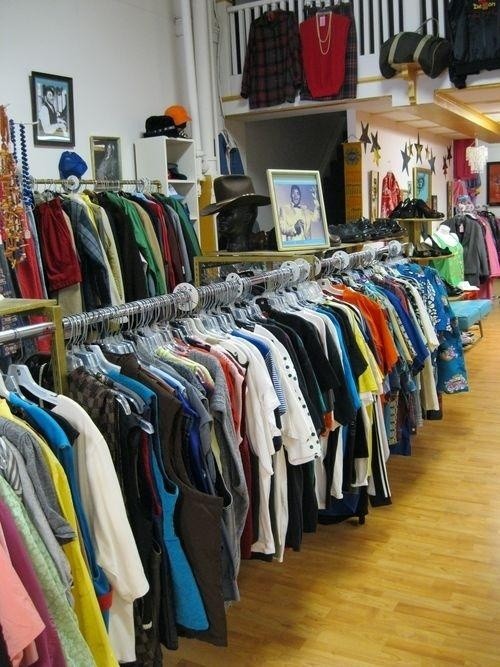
[450,299,493,351]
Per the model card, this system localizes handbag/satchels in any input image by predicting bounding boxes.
[380,30,449,78]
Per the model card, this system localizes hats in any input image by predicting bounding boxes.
[166,106,191,125]
[143,116,185,138]
[59,150,87,192]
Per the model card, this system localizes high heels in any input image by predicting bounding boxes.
[389,197,444,218]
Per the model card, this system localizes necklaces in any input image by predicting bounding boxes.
[313,11,334,55]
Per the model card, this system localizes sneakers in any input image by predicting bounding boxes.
[459,280,479,291]
[458,332,475,345]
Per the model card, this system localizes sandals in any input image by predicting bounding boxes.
[439,276,462,296]
[413,234,451,257]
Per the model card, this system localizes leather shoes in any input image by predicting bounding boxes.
[329,219,404,246]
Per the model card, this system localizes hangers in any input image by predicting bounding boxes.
[30,176,158,201]
[0,328,69,414]
[62,253,386,383]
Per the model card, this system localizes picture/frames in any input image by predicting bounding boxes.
[487,162,500,207]
[265,167,332,251]
[31,71,75,147]
[90,136,124,191]
[413,167,433,208]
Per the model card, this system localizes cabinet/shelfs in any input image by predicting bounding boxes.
[392,215,457,269]
[132,136,200,251]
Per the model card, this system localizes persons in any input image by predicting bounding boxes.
[96,142,119,187]
[277,182,322,241]
[39,86,67,135]
